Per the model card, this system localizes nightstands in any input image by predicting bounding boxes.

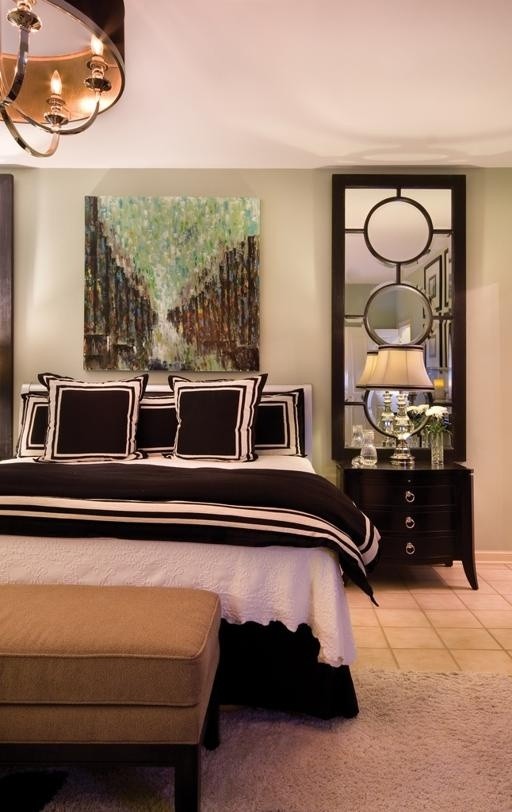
[336,462,480,592]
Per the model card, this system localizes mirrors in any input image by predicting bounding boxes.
[329,171,467,461]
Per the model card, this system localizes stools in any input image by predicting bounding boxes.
[3,580,222,811]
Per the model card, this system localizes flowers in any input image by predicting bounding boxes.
[424,406,453,439]
[405,404,427,434]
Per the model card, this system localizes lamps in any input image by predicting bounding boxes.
[357,351,396,461]
[3,0,126,159]
[367,344,432,464]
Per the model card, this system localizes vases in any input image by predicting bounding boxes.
[413,430,424,451]
[429,433,446,465]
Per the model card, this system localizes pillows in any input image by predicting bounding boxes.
[16,373,308,465]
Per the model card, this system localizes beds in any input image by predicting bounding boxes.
[0,377,358,726]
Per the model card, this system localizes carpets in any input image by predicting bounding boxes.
[36,668,510,811]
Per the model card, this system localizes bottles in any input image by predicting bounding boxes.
[350,423,367,450]
[360,431,379,467]
